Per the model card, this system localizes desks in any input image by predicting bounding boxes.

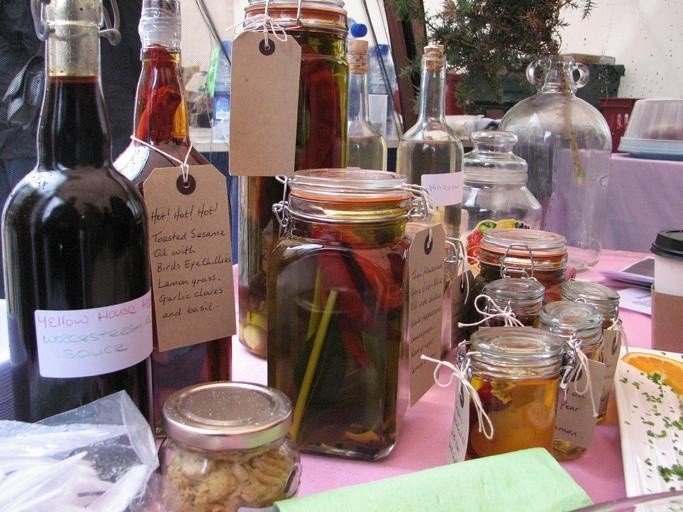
[135,250,681,511]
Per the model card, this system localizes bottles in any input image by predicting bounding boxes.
[266,170,411,462]
[465,327,565,454]
[162,379,301,512]
[535,299,607,460]
[0,0,156,446]
[559,280,625,424]
[212,38,231,144]
[350,20,396,170]
[233,0,349,358]
[115,0,234,471]
[495,54,615,274]
[483,279,548,330]
[461,131,543,278]
[396,43,462,357]
[463,228,571,338]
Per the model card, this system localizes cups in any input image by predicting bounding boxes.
[650,228,683,354]
